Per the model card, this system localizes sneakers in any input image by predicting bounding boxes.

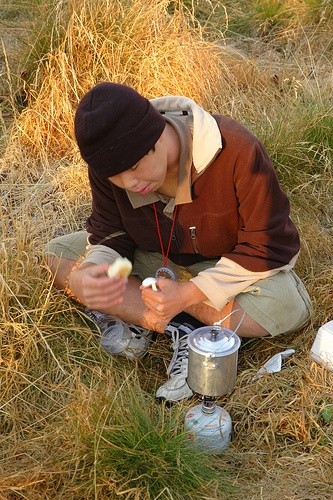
[116,323,153,361]
[153,337,196,404]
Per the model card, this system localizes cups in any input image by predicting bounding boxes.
[93,314,131,355]
[186,308,246,397]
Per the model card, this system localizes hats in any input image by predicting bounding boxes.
[73,81,165,181]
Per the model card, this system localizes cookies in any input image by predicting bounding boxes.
[107,257,131,280]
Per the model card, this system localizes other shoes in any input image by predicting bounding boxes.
[84,307,132,354]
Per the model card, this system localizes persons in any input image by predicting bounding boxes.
[42,82,313,409]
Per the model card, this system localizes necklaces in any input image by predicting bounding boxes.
[149,201,179,281]
